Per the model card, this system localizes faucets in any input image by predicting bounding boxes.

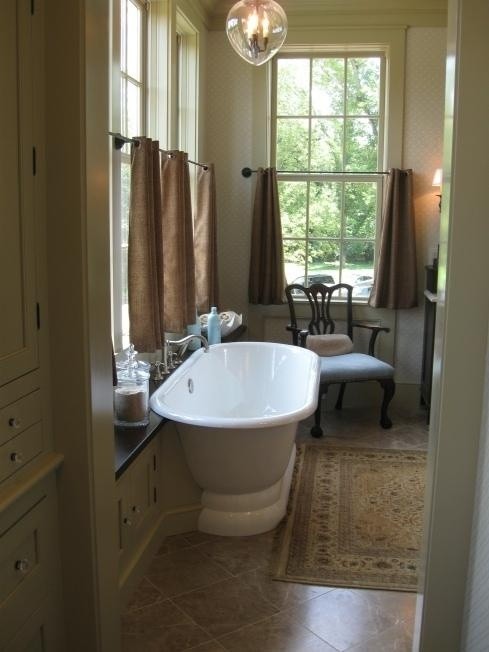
[161,334,210,378]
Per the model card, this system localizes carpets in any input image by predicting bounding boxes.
[271,439,428,594]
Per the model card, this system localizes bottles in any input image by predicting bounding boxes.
[112,343,151,426]
[186,306,221,351]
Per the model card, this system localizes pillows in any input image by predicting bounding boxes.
[306,333,355,357]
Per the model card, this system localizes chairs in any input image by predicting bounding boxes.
[284,282,397,438]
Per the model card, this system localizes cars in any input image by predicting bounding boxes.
[290,275,373,298]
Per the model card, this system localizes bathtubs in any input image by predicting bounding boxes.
[149,342,322,496]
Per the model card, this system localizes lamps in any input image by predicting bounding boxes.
[431,168,442,212]
[225,0,290,67]
